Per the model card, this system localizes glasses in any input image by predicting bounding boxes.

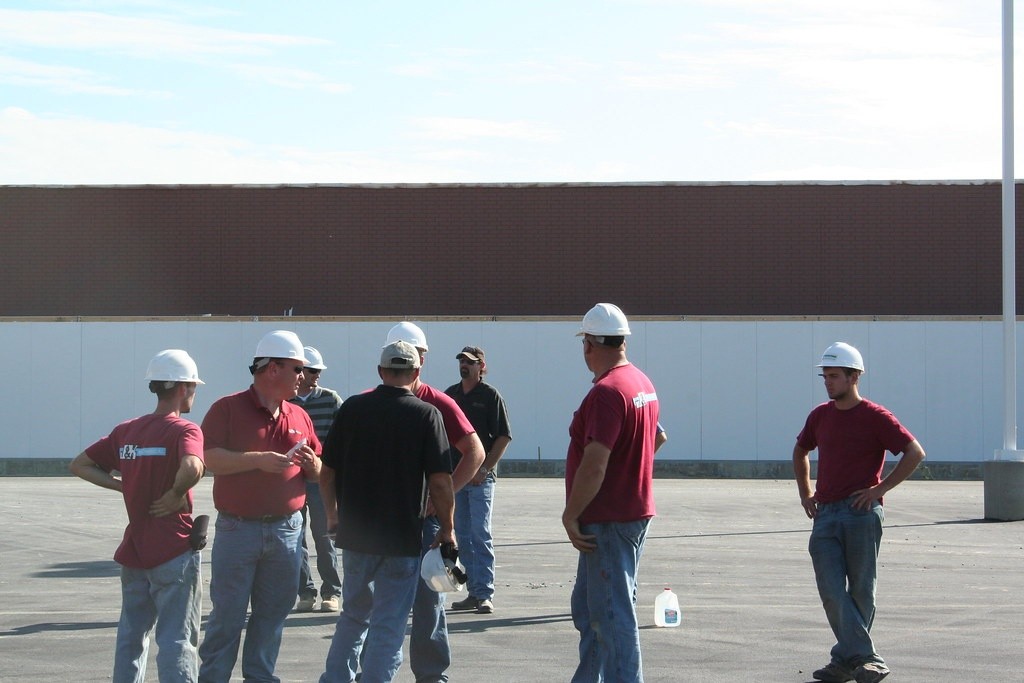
[459,358,480,365]
[304,366,321,374]
[277,362,304,374]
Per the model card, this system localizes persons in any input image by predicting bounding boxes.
[319,321,486,683]
[562,304,668,682]
[68,350,208,683]
[442,344,512,613]
[792,340,926,682]
[284,347,344,612]
[200,329,323,683]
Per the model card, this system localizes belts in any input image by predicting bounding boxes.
[242,515,287,523]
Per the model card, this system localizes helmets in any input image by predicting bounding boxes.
[574,303,631,336]
[303,346,327,369]
[254,330,311,365]
[421,546,462,592]
[381,322,428,352]
[145,349,205,385]
[816,342,865,371]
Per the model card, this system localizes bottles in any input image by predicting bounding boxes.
[654,588,681,627]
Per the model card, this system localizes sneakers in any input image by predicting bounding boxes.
[853,662,890,683]
[813,664,855,683]
[321,595,339,611]
[452,597,477,610]
[477,600,493,613]
[297,593,316,611]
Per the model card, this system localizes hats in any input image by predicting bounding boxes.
[380,340,420,368]
[190,515,210,550]
[456,346,485,363]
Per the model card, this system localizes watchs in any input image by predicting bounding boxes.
[479,465,488,475]
